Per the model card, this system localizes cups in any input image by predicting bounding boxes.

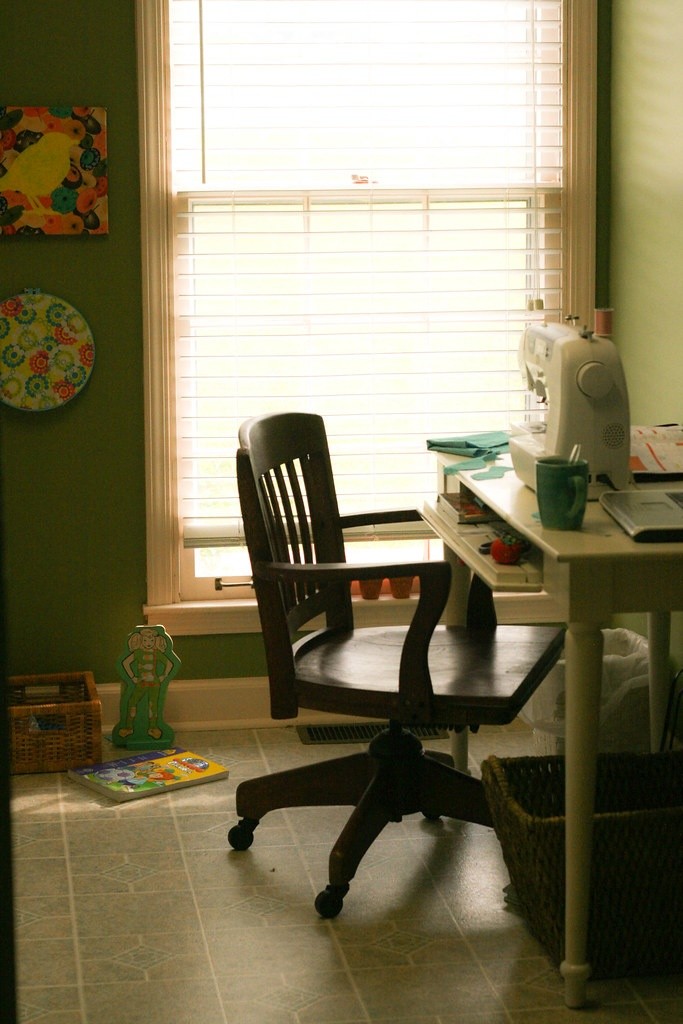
[535,456,589,531]
[388,577,414,599]
[359,579,383,600]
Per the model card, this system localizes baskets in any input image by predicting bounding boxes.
[7,671,103,775]
[481,751,683,980]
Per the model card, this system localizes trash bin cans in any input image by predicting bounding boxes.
[529,625,651,755]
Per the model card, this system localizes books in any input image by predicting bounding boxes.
[437,492,502,525]
[67,746,229,803]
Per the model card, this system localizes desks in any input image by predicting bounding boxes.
[416,451,683,1010]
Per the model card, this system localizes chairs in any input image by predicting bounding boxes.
[229,412,567,921]
